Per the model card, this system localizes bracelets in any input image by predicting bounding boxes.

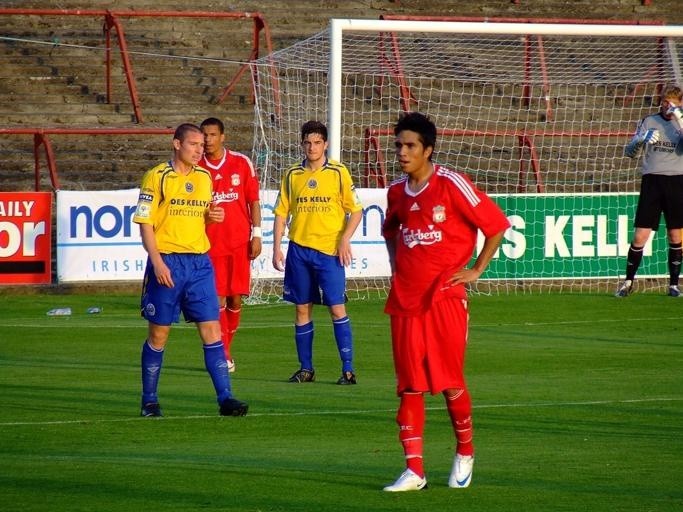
[252,225,261,238]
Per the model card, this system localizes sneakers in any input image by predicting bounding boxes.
[337,373,357,385]
[142,396,162,416]
[382,468,429,492]
[225,359,236,373]
[449,452,474,488]
[668,285,683,297]
[615,280,634,297]
[289,368,315,383]
[220,399,248,416]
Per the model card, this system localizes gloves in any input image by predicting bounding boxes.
[666,102,683,130]
[637,128,659,144]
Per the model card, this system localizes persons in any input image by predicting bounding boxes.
[132,123,252,416]
[381,113,510,491]
[198,117,263,371]
[616,86,682,298]
[271,121,363,385]
